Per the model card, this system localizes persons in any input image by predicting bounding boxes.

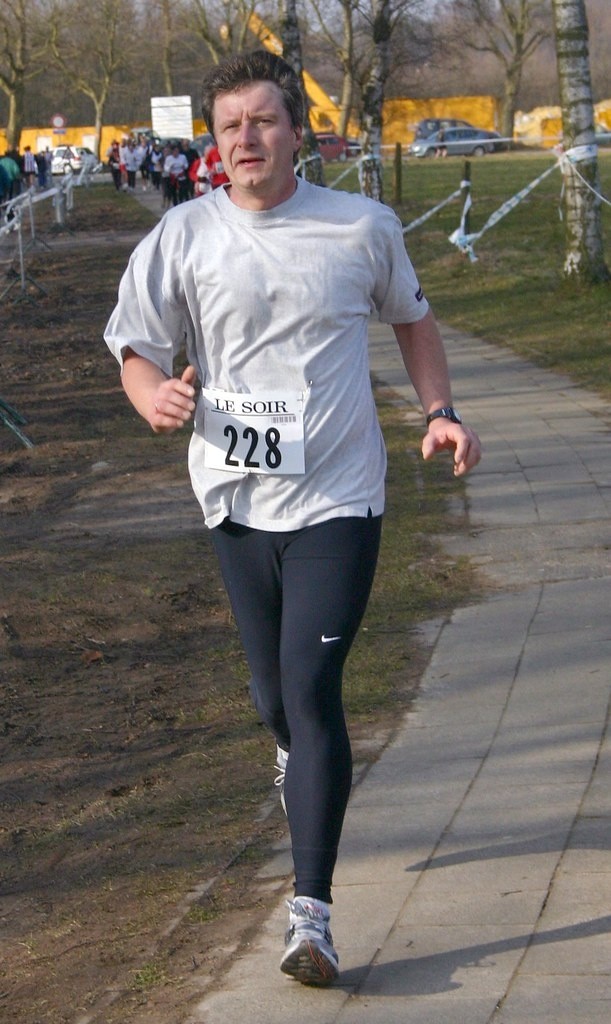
[436,128,448,157]
[102,52,481,983]
[108,130,229,207]
[0,146,73,235]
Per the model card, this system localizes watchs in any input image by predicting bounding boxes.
[424,407,463,425]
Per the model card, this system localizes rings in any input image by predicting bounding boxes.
[154,400,159,412]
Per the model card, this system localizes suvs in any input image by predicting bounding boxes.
[413,119,508,152]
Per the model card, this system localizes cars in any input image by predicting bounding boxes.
[315,132,362,162]
[159,133,218,158]
[49,147,98,175]
[408,127,494,159]
[595,122,611,146]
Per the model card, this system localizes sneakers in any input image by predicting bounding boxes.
[279,899,340,985]
[274,747,288,815]
